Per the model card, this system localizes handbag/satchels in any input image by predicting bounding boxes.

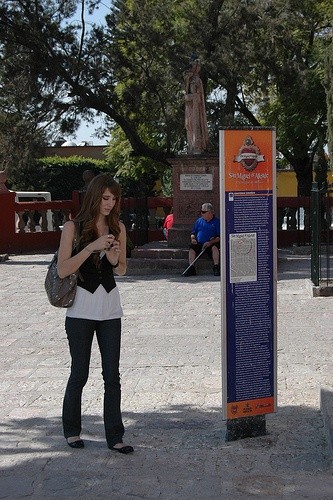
[45,220,79,308]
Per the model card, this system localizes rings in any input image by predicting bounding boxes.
[107,234,110,238]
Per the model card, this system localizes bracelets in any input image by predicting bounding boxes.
[111,260,120,268]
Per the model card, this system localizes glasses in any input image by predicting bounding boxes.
[200,209,210,214]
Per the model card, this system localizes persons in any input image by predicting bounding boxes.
[182,59,209,155]
[162,206,174,242]
[183,203,220,276]
[57,174,134,454]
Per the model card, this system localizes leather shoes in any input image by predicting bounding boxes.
[66,436,84,448]
[108,443,134,453]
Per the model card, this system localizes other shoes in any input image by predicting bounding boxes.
[213,265,220,276]
[184,267,196,276]
